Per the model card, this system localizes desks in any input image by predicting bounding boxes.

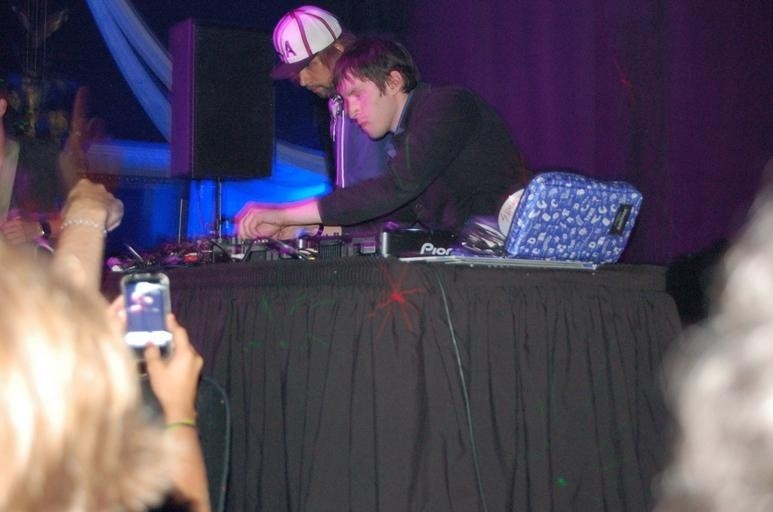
[100,260,667,511]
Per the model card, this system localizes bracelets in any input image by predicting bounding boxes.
[310,223,324,239]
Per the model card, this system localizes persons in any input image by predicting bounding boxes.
[1,81,213,511]
[259,9,417,240]
[233,35,524,247]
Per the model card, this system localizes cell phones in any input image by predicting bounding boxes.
[120,272,170,346]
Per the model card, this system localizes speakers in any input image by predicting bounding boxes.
[173,18,277,179]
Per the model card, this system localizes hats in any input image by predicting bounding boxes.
[272,5,342,80]
